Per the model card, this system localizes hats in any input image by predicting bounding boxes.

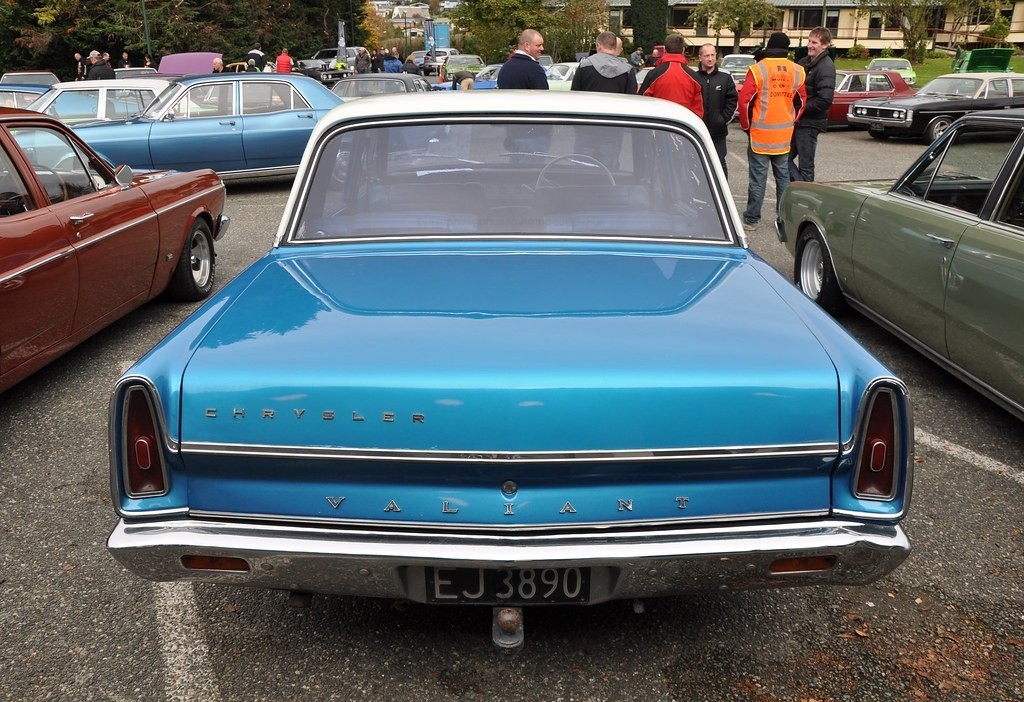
[637,47,643,53]
[282,48,288,52]
[87,50,101,59]
[380,46,385,50]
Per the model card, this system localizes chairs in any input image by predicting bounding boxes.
[368,182,485,209]
[535,184,647,219]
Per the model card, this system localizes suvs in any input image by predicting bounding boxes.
[298,47,371,74]
[423,48,462,76]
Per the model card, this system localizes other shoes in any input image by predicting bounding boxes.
[739,217,757,231]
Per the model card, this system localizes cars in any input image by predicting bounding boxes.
[440,54,487,82]
[432,63,504,91]
[544,62,581,90]
[0,72,61,109]
[774,108,1024,419]
[846,72,1024,144]
[827,70,919,125]
[113,53,353,104]
[13,71,353,182]
[106,88,915,651]
[537,54,552,69]
[720,54,757,83]
[26,79,202,126]
[411,51,429,68]
[331,73,432,104]
[865,58,917,87]
[0,107,230,395]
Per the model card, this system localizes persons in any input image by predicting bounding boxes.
[735,32,808,233]
[788,26,836,184]
[498,27,738,183]
[74,43,476,92]
